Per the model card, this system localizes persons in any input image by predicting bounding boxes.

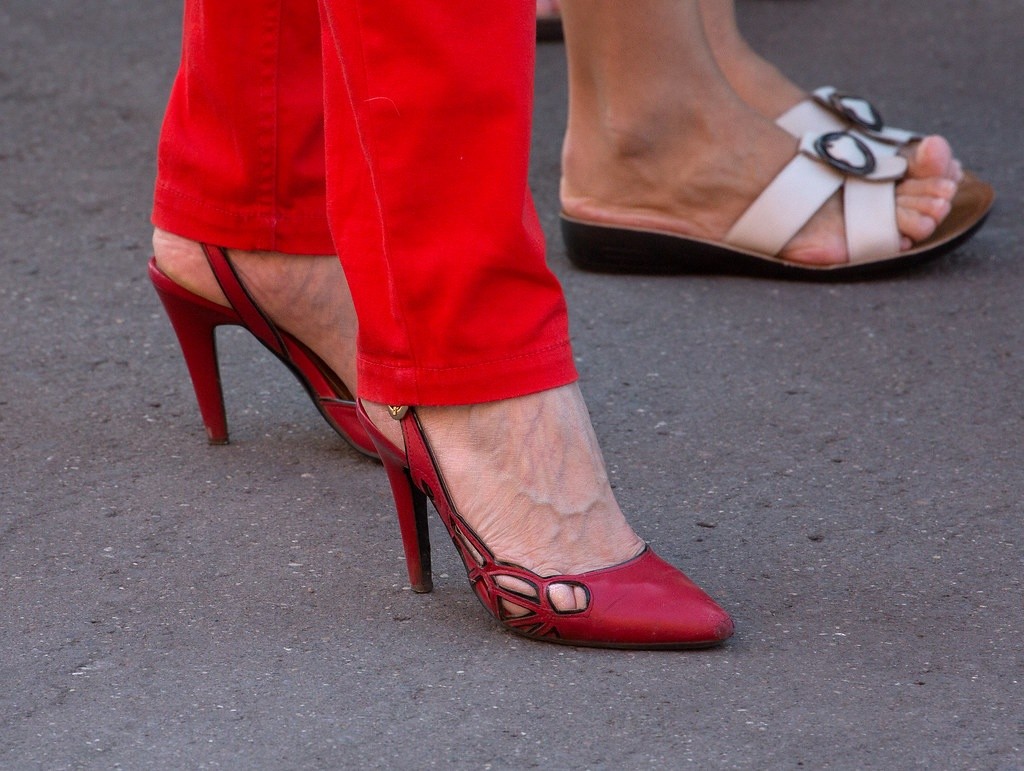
[149,1,732,650]
[557,0,996,282]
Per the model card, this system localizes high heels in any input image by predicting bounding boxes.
[355,396,734,651]
[146,237,384,460]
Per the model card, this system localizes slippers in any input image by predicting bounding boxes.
[770,86,980,182]
[560,129,995,283]
